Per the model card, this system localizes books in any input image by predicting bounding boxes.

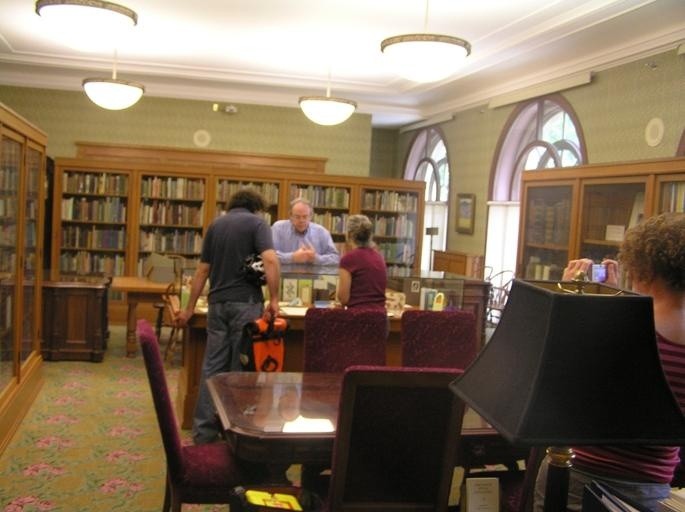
[263,276,339,316]
[525,199,572,281]
[0,136,35,328]
[61,171,129,282]
[365,190,417,275]
[217,180,278,225]
[670,182,685,214]
[384,286,445,317]
[182,276,208,312]
[290,184,348,253]
[138,177,204,277]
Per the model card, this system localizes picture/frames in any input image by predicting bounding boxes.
[454,192,475,236]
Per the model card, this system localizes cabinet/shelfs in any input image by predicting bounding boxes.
[0,100,47,461]
[135,159,210,328]
[210,161,286,225]
[356,175,426,281]
[50,156,136,326]
[576,158,684,264]
[515,156,581,284]
[287,173,357,261]
[434,248,485,278]
[43,279,110,362]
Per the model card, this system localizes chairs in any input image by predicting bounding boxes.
[135,318,293,512]
[303,307,388,372]
[311,364,466,511]
[401,309,479,370]
[497,443,542,510]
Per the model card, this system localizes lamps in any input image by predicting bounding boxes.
[374,4,470,81]
[299,64,356,128]
[82,52,145,112]
[426,226,439,273]
[35,0,139,45]
[445,278,683,511]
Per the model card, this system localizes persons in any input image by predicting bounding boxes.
[272,198,341,266]
[171,186,282,445]
[561,210,685,506]
[338,214,387,309]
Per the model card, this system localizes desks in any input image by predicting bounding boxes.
[165,292,418,429]
[111,275,180,358]
[206,369,530,512]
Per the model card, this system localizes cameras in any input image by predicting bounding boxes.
[588,265,607,282]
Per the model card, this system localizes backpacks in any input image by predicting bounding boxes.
[239,312,289,376]
